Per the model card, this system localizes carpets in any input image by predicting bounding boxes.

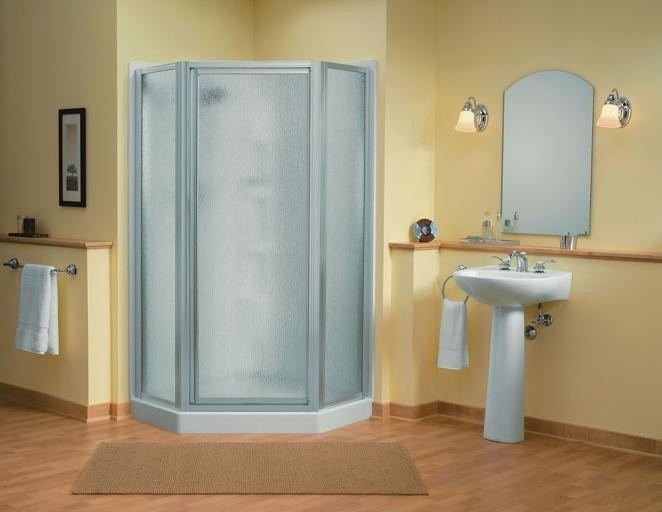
[69,440,430,498]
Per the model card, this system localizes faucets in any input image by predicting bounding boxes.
[511,249,529,272]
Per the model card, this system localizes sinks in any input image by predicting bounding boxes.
[453,265,572,309]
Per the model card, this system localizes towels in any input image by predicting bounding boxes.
[435,298,473,372]
[13,260,63,356]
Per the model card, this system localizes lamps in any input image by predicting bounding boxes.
[453,93,490,137]
[594,87,631,130]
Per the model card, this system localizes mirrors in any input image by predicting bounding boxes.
[499,67,597,239]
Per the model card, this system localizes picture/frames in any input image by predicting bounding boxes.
[56,105,88,210]
